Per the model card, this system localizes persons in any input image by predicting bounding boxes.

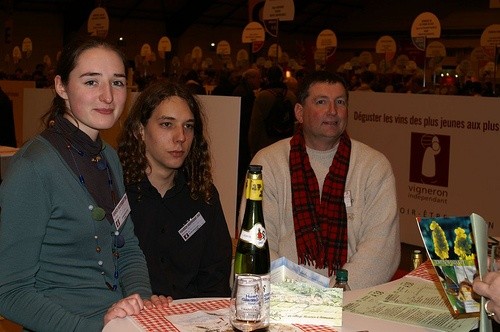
[472,270,500,325]
[0,37,174,330]
[111,80,234,299]
[237,68,402,294]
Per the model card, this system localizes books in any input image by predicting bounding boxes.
[415,212,489,317]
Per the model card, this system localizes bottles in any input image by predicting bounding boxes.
[411,250,421,272]
[332,270,350,291]
[234,165,270,332]
